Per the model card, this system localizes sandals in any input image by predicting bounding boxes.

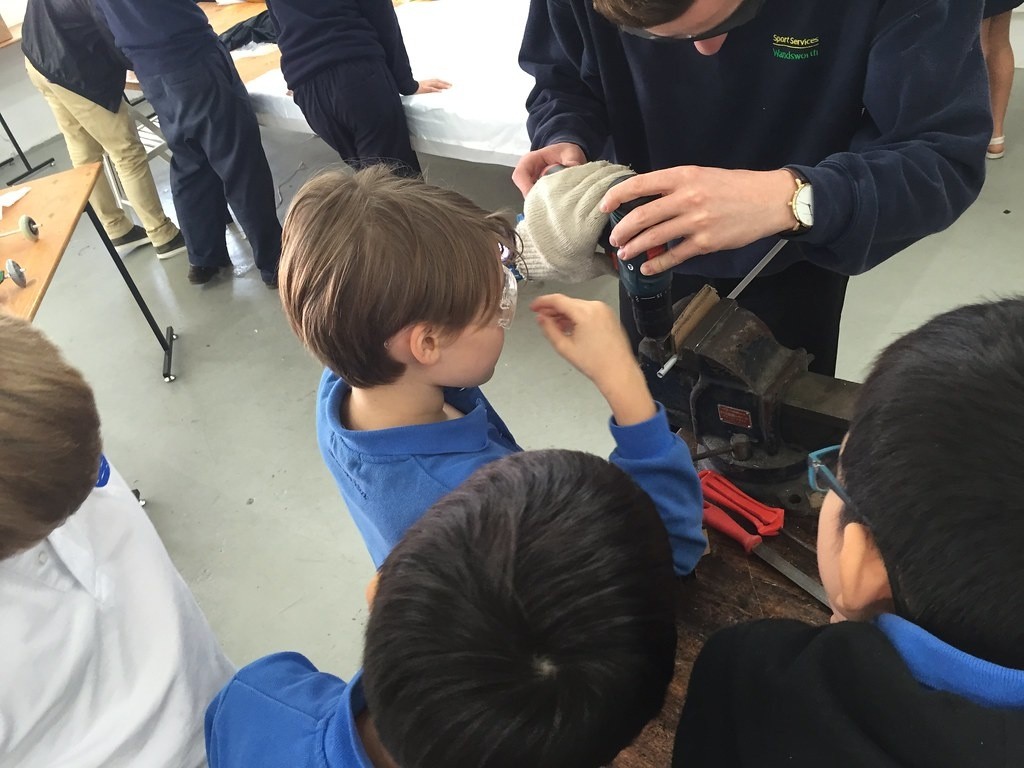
[985,135,1005,159]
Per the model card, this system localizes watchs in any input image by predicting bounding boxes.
[782,167,814,239]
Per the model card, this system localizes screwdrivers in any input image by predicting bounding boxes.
[697,470,817,554]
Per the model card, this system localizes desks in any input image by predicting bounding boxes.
[0,161,180,384]
[240,0,535,172]
[0,0,269,187]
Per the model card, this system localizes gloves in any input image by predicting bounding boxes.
[512,161,636,284]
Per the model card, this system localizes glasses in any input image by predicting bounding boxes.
[806,445,911,625]
[617,0,763,42]
[383,239,519,351]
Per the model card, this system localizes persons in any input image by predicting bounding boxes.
[672,298,1023,768]
[201,448,679,768]
[978,0,1022,159]
[22,0,186,261]
[92,0,284,291]
[0,318,233,768]
[276,165,702,580]
[266,0,451,182]
[509,1,992,376]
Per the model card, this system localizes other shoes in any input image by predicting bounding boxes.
[188,265,219,284]
[154,230,187,260]
[110,225,151,252]
[260,272,278,289]
